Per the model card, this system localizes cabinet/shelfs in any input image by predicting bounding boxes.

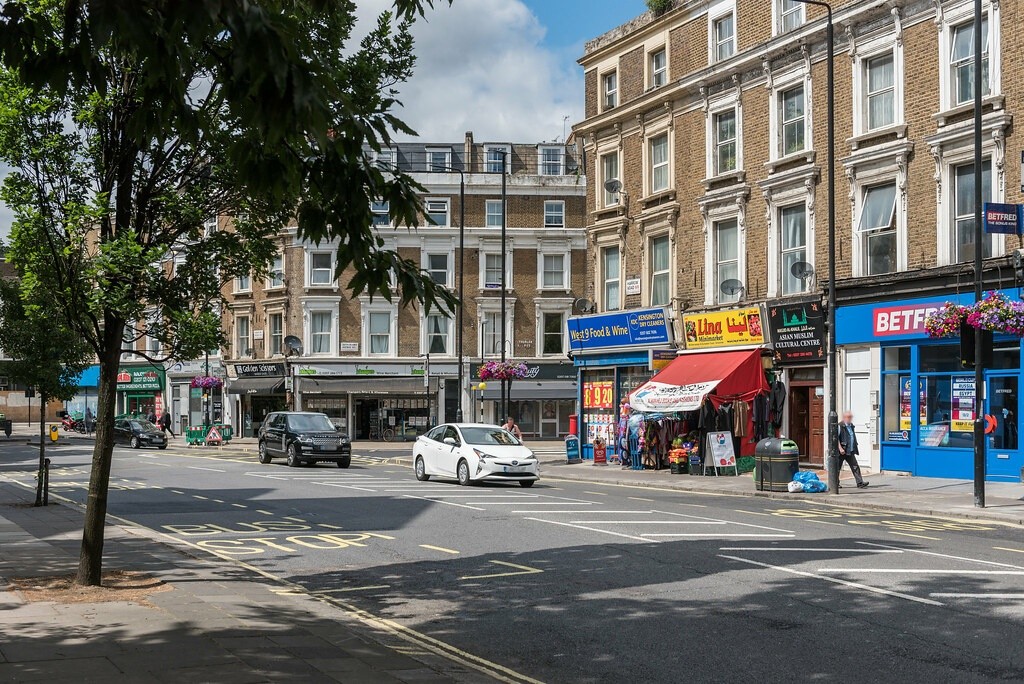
[619,374,652,400]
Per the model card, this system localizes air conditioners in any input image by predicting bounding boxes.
[311,268,332,286]
[371,200,390,215]
[428,203,447,212]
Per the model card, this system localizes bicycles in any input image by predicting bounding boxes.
[368,424,395,442]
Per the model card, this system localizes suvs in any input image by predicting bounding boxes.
[257,410,352,469]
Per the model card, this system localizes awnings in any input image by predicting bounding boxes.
[625,349,773,412]
[228,378,285,395]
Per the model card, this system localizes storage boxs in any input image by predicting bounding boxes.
[667,445,703,476]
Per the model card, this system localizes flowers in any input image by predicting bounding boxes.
[191,374,223,388]
[925,289,1024,339]
[477,358,530,381]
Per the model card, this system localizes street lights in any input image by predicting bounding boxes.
[175,239,211,426]
[427,164,465,424]
[789,0,841,496]
[480,319,490,382]
[489,148,508,425]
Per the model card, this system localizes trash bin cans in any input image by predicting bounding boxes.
[754,436,800,492]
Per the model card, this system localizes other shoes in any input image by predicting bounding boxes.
[857,482,869,488]
[837,485,842,488]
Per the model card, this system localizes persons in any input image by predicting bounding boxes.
[147,409,157,425]
[501,417,523,444]
[1004,411,1018,450]
[160,409,175,439]
[542,401,556,419]
[521,403,531,422]
[837,413,869,489]
[84,407,93,438]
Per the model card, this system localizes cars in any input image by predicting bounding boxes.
[112,418,170,450]
[411,421,541,488]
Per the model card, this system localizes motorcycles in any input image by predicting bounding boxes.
[56,410,98,434]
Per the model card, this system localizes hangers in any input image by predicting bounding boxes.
[723,396,742,402]
